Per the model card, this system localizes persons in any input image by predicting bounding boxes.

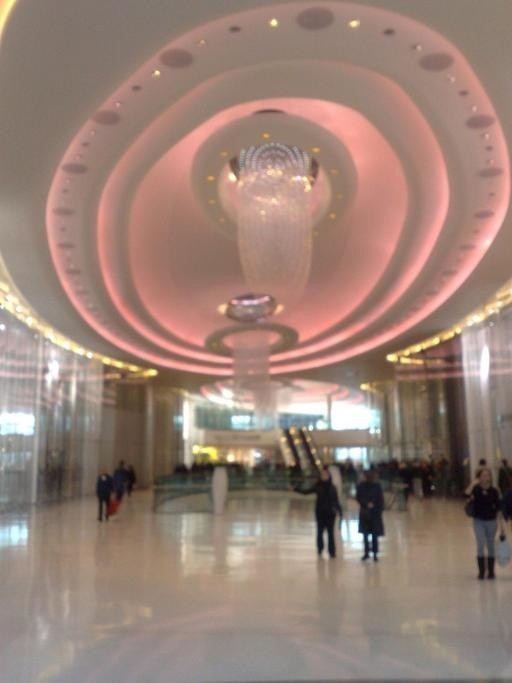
[356,470,385,561]
[498,459,512,520]
[340,454,463,503]
[113,460,130,501]
[174,458,305,486]
[288,470,342,557]
[128,464,136,496]
[96,465,114,522]
[475,459,491,479]
[464,468,506,579]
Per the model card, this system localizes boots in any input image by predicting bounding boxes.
[477,556,496,580]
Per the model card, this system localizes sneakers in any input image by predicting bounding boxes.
[361,553,380,562]
[317,549,339,558]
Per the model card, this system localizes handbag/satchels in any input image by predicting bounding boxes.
[464,496,479,517]
[497,530,510,568]
